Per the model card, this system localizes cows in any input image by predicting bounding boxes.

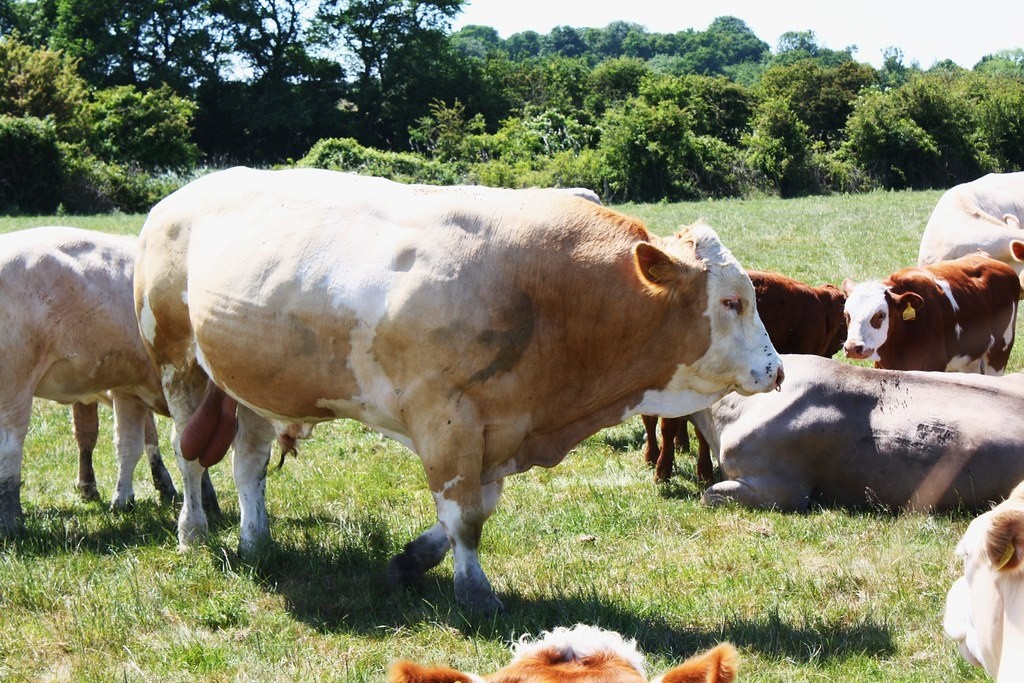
[0,166,1024,682]
